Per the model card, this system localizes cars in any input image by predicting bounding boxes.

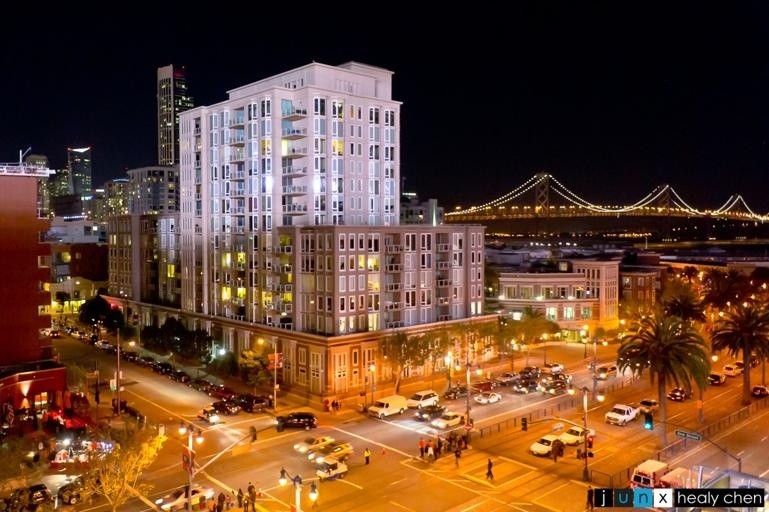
[0,468,117,512]
[293,433,355,481]
[154,482,214,510]
[40,323,269,423]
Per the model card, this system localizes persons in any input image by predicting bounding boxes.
[419,431,467,468]
[552,444,559,462]
[486,458,494,480]
[364,448,371,464]
[207,481,256,512]
[332,399,342,410]
[31,411,97,465]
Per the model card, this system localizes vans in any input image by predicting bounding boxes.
[274,411,319,432]
[368,395,409,420]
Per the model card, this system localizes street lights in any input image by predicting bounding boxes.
[279,466,318,512]
[178,419,203,512]
[568,383,606,482]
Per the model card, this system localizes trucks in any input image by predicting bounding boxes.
[629,459,712,488]
